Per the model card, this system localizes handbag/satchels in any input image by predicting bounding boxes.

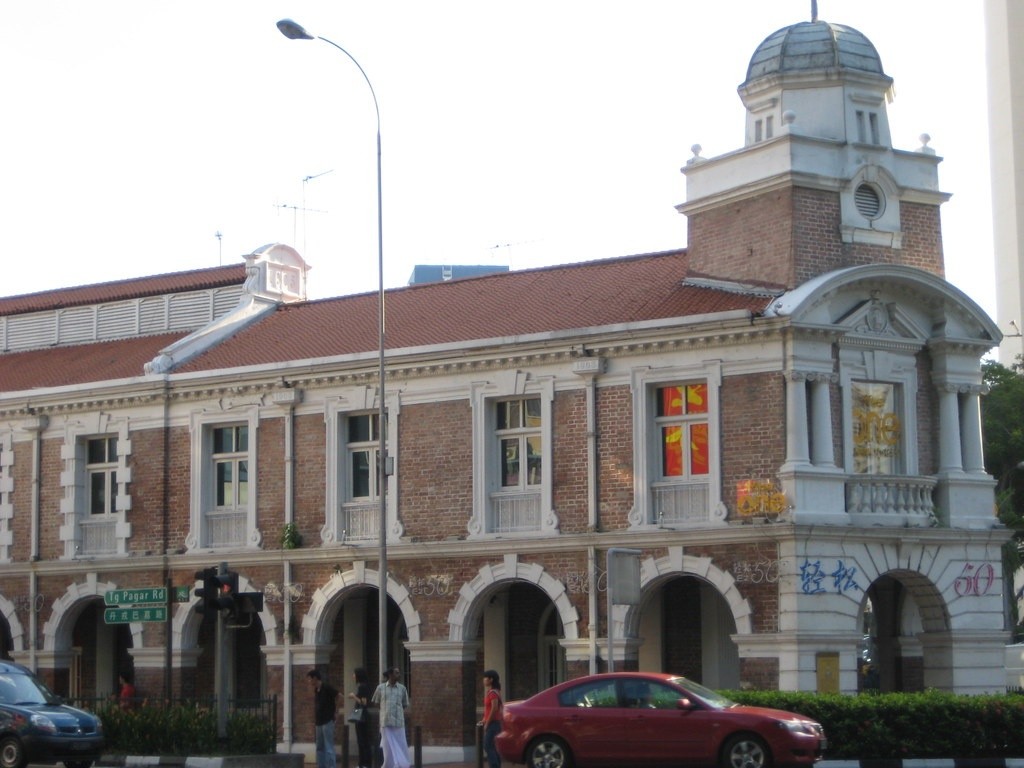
[347,698,368,724]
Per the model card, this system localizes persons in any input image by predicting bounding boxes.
[479,669,503,768]
[119,671,136,710]
[638,684,656,709]
[306,666,410,768]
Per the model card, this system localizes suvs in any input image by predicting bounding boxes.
[0,659,106,768]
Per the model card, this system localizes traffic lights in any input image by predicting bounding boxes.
[194,567,219,621]
[210,571,241,618]
[237,591,264,613]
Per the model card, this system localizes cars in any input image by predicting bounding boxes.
[495,671,826,768]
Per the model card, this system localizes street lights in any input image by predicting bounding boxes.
[276,15,390,685]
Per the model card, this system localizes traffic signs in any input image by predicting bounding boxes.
[103,606,168,625]
[103,586,168,606]
